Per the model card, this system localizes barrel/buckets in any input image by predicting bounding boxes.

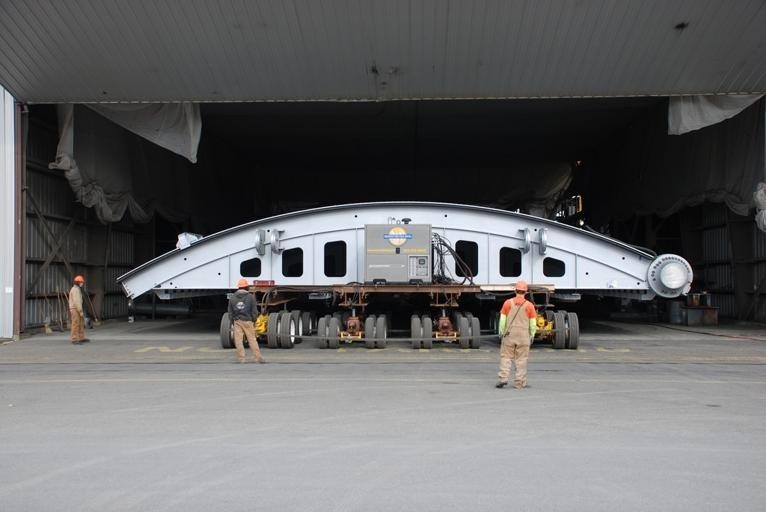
[686,292,701,306]
[669,300,683,324]
[701,292,712,307]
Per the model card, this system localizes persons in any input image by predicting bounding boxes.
[228,279,268,363]
[68,276,90,345]
[496,282,538,389]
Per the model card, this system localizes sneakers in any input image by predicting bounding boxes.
[72,338,90,345]
[496,382,508,389]
[256,359,269,364]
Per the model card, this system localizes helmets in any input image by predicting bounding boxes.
[516,280,528,291]
[73,276,85,283]
[236,278,250,288]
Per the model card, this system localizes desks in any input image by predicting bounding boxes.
[680,305,720,326]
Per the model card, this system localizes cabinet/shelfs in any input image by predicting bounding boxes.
[668,300,719,324]
